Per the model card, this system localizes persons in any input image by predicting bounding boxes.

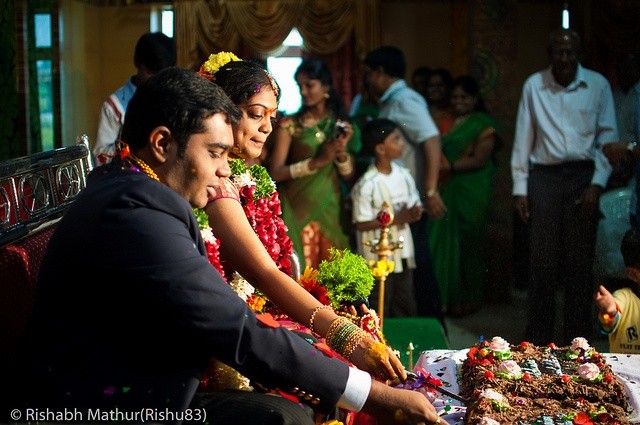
[191,52,408,424]
[350,119,422,319]
[22,67,449,425]
[425,69,453,177]
[91,32,178,166]
[360,47,449,340]
[268,58,357,274]
[510,28,590,345]
[423,76,500,317]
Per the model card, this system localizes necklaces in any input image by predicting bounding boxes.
[114,139,160,179]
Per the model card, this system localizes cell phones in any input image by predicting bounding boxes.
[332,122,347,140]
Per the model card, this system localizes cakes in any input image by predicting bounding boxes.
[458,337,638,424]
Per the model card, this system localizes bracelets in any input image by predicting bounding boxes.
[310,304,369,362]
[337,153,357,177]
[334,156,353,178]
[290,157,319,179]
[422,189,439,199]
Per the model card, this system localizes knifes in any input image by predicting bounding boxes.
[405,370,470,405]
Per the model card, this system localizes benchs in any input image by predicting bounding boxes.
[1,132,98,348]
